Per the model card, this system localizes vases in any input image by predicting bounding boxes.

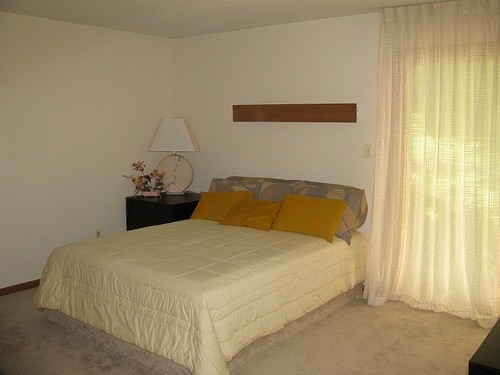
[141,191,158,196]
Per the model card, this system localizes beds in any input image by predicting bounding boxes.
[34,178,369,375]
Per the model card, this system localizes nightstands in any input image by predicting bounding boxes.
[126,192,201,231]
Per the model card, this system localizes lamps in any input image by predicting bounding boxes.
[149,117,200,194]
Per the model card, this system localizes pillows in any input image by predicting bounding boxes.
[191,177,364,246]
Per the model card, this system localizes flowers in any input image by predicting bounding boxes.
[123,163,167,198]
